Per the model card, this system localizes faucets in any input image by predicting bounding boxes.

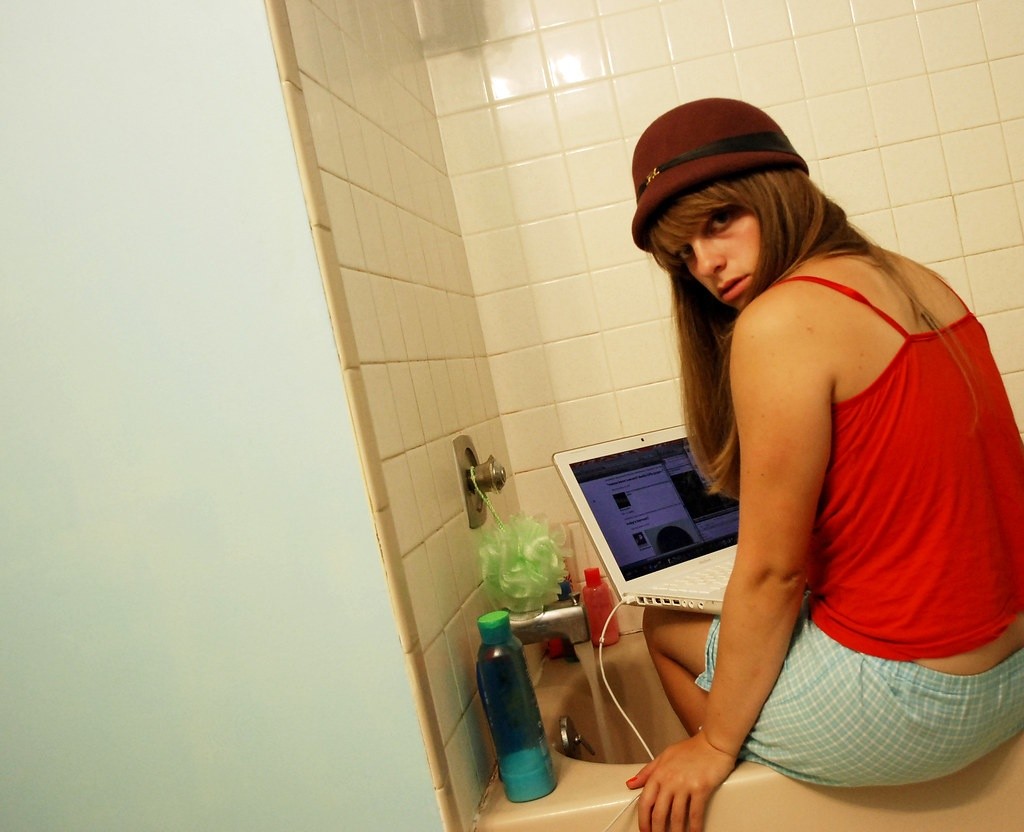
[490,604,591,645]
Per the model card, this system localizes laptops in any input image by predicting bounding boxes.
[552,423,812,615]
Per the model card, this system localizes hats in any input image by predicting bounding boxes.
[632,98,809,251]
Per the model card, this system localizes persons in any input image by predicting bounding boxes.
[625,96,1024,832]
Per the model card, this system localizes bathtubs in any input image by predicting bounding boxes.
[475,595,1023,830]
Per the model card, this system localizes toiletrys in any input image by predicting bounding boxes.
[474,610,560,805]
[525,516,622,665]
